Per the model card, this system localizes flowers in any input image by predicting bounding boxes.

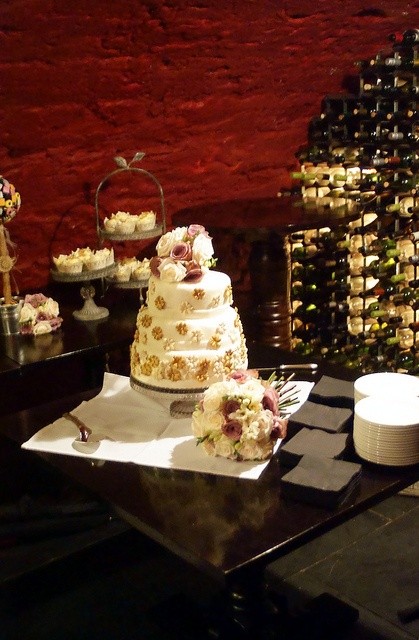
[14,293,63,339]
[191,370,302,462]
[149,224,219,283]
[0,174,21,225]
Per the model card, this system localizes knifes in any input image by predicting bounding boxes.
[254,363,318,371]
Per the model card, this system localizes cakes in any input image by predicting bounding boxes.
[114,257,152,283]
[103,210,157,233]
[52,246,114,274]
[130,269,249,390]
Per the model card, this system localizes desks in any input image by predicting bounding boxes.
[0,286,139,379]
[171,197,361,349]
[1,341,419,610]
[265,480,419,640]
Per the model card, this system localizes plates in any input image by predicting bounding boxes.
[352,372,419,467]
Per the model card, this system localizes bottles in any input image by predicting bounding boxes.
[388,31,404,44]
[403,28,419,43]
[370,58,385,68]
[276,81,419,375]
[352,60,369,69]
[400,57,419,76]
[384,56,413,72]
